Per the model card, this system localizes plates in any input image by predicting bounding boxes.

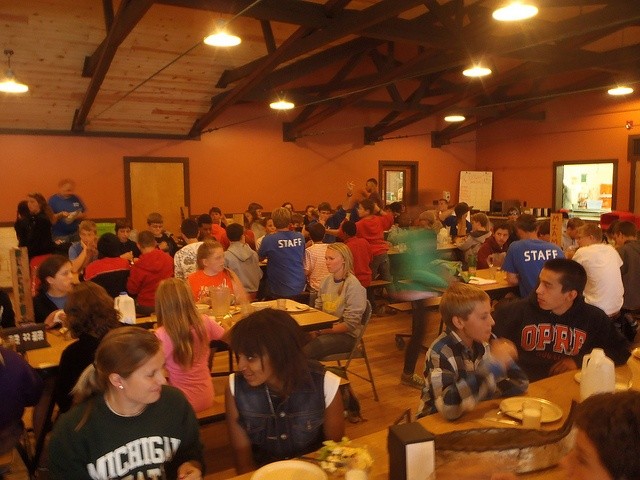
[250,302,272,311]
[498,396,563,423]
[575,371,632,392]
[285,304,308,312]
[247,459,326,480]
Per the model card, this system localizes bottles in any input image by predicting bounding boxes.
[468,254,476,278]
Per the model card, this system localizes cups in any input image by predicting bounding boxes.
[210,287,237,319]
[522,401,542,431]
[488,266,497,279]
[499,270,507,286]
[278,298,286,311]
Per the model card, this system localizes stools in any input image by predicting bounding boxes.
[205,341,242,375]
[1,421,33,474]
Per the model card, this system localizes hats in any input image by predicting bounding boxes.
[455,201,474,216]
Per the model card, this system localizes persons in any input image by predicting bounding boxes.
[45,325,206,480]
[14,201,32,247]
[610,222,639,314]
[356,199,398,316]
[258,207,305,299]
[27,193,53,257]
[443,203,473,237]
[209,207,228,229]
[386,187,453,390]
[69,220,99,271]
[83,233,131,300]
[415,283,530,421]
[562,218,583,253]
[571,224,624,317]
[538,222,550,242]
[48,180,86,254]
[248,203,263,219]
[257,218,276,251]
[561,391,639,480]
[438,198,453,220]
[153,277,232,412]
[420,211,440,234]
[245,210,264,241]
[282,202,293,212]
[0,288,16,328]
[454,212,492,263]
[401,204,406,213]
[503,213,565,299]
[197,214,218,242]
[52,281,122,414]
[115,219,141,260]
[127,231,174,315]
[304,223,331,294]
[303,205,317,225]
[291,213,304,232]
[477,223,512,268]
[222,223,263,302]
[187,241,248,308]
[175,217,207,280]
[305,243,368,361]
[224,308,345,476]
[390,202,402,218]
[342,221,373,288]
[31,253,80,325]
[307,202,346,243]
[244,216,256,250]
[508,207,520,221]
[137,212,179,256]
[0,305,44,455]
[493,259,632,383]
[360,178,382,215]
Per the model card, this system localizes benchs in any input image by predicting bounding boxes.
[383,294,446,351]
[169,367,351,422]
[290,274,394,304]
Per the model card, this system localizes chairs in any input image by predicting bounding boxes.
[316,294,383,408]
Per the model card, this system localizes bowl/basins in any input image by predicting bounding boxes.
[196,303,210,314]
